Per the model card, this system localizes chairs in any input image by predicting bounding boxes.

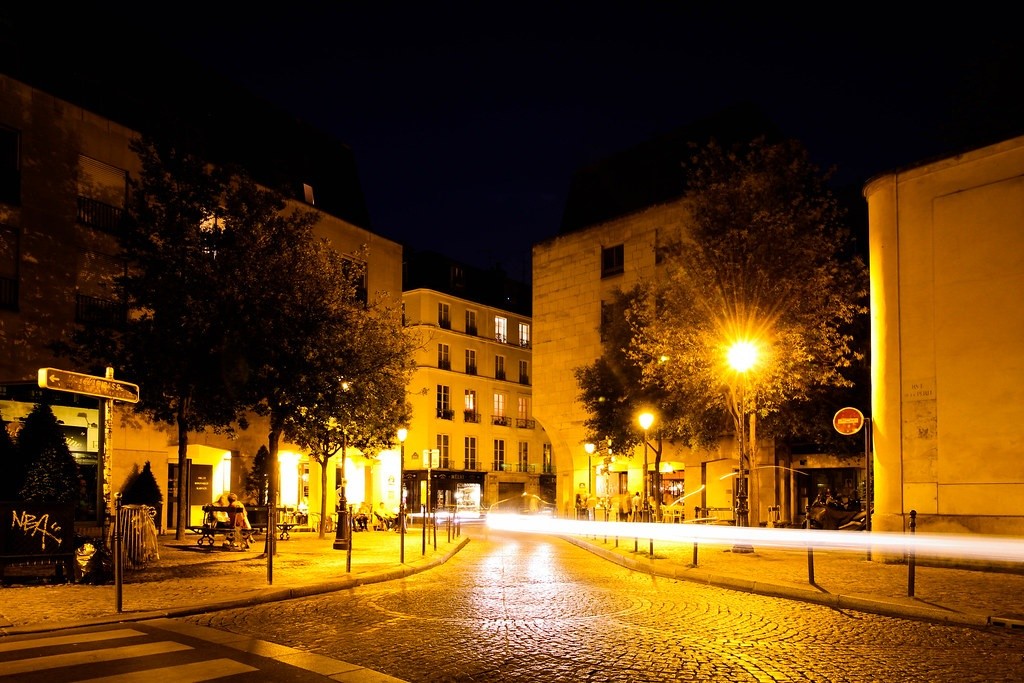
[652,503,684,524]
[374,510,390,532]
[310,512,333,533]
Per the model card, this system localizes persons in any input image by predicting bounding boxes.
[295,496,309,524]
[227,493,255,549]
[211,493,238,548]
[576,491,655,522]
[352,502,371,532]
[812,488,861,511]
[377,502,397,532]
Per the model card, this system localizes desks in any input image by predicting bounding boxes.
[330,512,338,532]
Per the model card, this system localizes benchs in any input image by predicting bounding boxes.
[691,505,736,526]
[185,506,258,552]
[247,507,298,541]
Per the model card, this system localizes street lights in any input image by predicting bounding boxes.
[585,442,595,520]
[726,340,757,553]
[639,412,655,524]
[397,427,406,562]
[332,377,352,550]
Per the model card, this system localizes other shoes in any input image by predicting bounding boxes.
[366,529,369,532]
[251,540,255,543]
[361,527,365,531]
[223,543,229,546]
[246,545,249,548]
[388,529,392,532]
[228,545,239,547]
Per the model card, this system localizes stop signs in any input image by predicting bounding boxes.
[833,409,864,434]
